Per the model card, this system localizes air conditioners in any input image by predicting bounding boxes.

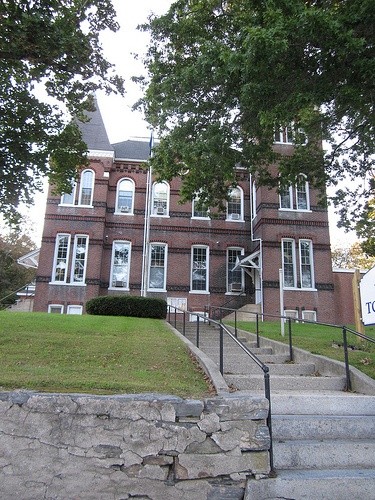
[115,281,123,287]
[157,207,164,214]
[231,213,239,220]
[120,206,129,212]
[230,283,243,291]
